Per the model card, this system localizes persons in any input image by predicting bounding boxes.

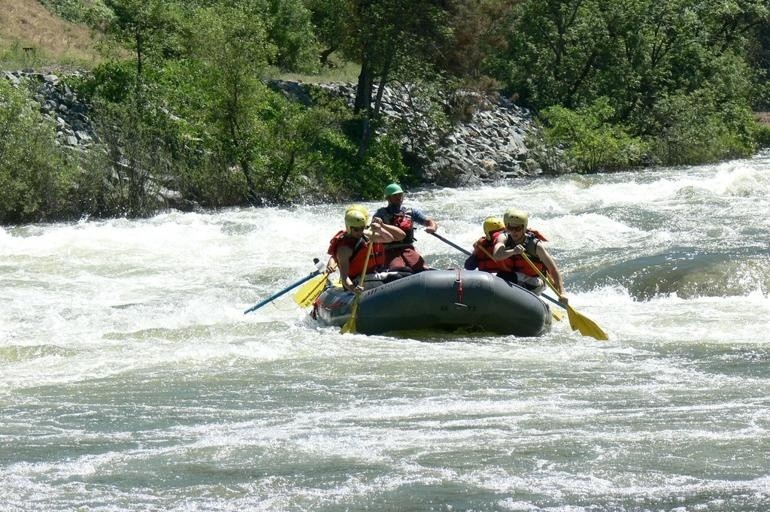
[324,204,413,280]
[370,184,439,274]
[464,215,506,273]
[494,208,570,305]
[335,210,403,294]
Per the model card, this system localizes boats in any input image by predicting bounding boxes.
[313,267,553,339]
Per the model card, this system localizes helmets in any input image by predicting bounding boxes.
[346,203,369,223]
[384,184,404,199]
[482,217,504,242]
[503,208,528,233]
[344,210,366,229]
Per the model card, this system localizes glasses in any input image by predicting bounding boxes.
[508,225,522,233]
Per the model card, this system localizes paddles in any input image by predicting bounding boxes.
[521,251,608,342]
[340,230,377,335]
[294,261,340,310]
